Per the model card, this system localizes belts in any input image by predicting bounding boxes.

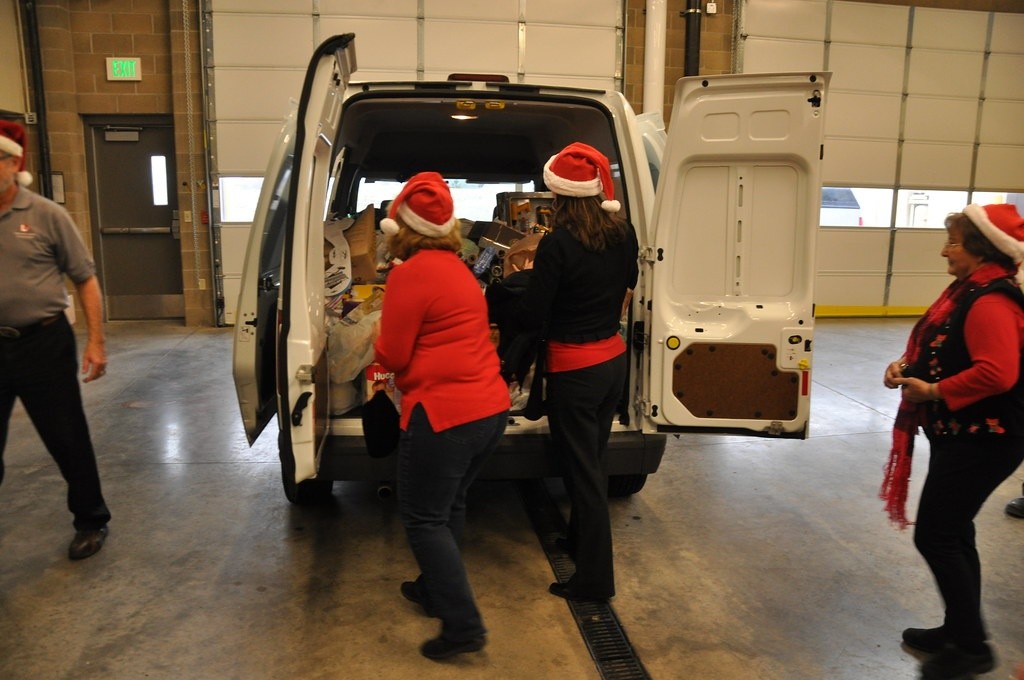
[0,312,64,337]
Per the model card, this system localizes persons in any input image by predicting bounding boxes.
[372,173,514,660]
[879,203,1024,680]
[489,141,641,601]
[0,116,113,560]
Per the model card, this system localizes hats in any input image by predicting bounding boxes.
[0,117,33,187]
[380,172,455,239]
[964,203,1024,264]
[543,145,622,214]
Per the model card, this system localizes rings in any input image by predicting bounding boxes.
[99,371,106,375]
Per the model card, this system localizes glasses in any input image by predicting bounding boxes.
[944,239,971,253]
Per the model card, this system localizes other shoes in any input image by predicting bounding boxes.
[921,643,992,680]
[902,624,959,655]
[69,522,108,558]
[551,577,610,602]
[1005,497,1024,518]
[422,630,488,659]
[402,581,438,617]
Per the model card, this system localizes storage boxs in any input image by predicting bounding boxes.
[361,363,395,404]
[466,221,525,250]
[323,203,378,284]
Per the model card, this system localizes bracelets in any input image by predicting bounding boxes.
[930,383,935,399]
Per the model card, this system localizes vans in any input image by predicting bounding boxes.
[232,31,833,507]
